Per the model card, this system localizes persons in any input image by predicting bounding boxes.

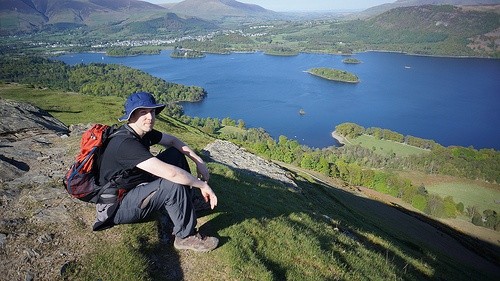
[96,92,219,252]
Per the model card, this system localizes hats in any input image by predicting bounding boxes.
[118,91,167,122]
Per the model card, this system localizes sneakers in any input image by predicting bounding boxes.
[173,232,218,252]
[156,225,174,241]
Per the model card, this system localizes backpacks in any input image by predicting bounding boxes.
[63,123,142,203]
